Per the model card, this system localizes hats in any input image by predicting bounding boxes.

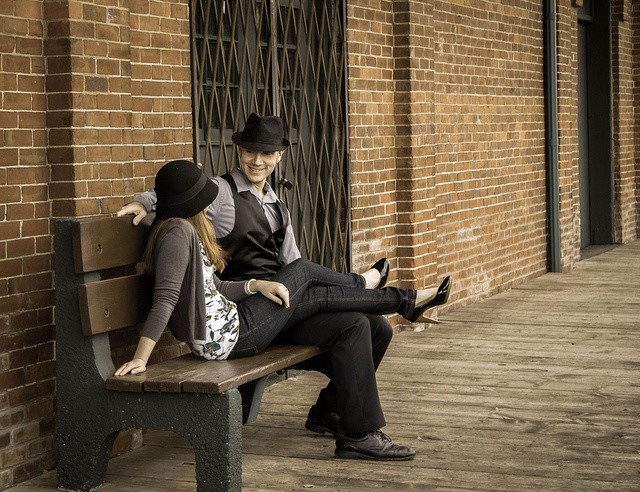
[232,112,290,151]
[154,160,218,221]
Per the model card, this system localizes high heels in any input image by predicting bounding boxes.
[370,258,389,290]
[407,276,451,324]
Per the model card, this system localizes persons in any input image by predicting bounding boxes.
[118,113,415,460]
[113,160,452,376]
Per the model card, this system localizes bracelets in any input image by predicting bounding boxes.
[247,277,256,296]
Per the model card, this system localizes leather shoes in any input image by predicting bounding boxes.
[304,406,340,437]
[335,428,416,461]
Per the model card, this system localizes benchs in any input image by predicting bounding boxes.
[53,212,334,492]
